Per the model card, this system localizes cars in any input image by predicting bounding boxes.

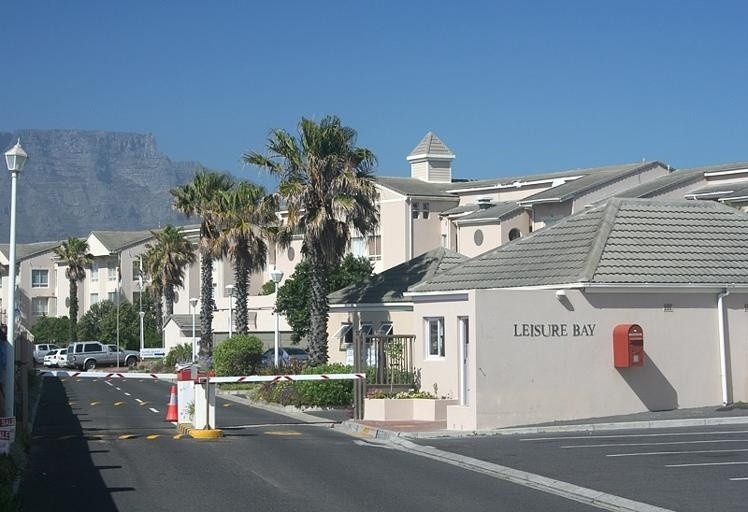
[260,346,313,369]
[43,348,68,368]
[58,348,75,369]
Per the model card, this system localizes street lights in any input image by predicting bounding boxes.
[270,269,285,371]
[3,136,29,456]
[139,311,145,360]
[189,297,199,362]
[225,284,235,339]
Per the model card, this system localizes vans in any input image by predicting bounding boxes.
[32,343,63,364]
[67,340,112,369]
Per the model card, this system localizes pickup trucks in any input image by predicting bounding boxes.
[67,343,140,371]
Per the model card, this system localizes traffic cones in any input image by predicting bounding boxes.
[163,385,178,422]
